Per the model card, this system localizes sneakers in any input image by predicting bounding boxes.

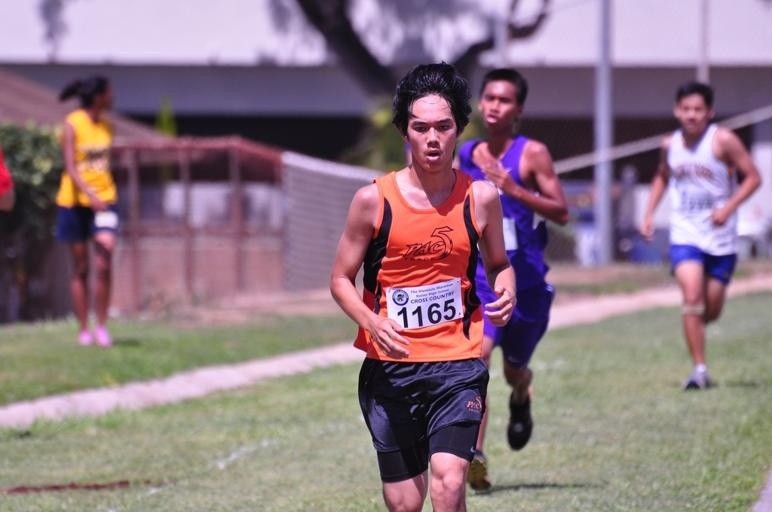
[464,451,494,497]
[75,324,113,348]
[506,390,533,450]
[684,370,711,389]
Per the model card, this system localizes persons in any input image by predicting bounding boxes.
[642,82,762,389]
[53,74,117,344]
[456,69,569,492]
[329,63,518,511]
[1,148,14,211]
